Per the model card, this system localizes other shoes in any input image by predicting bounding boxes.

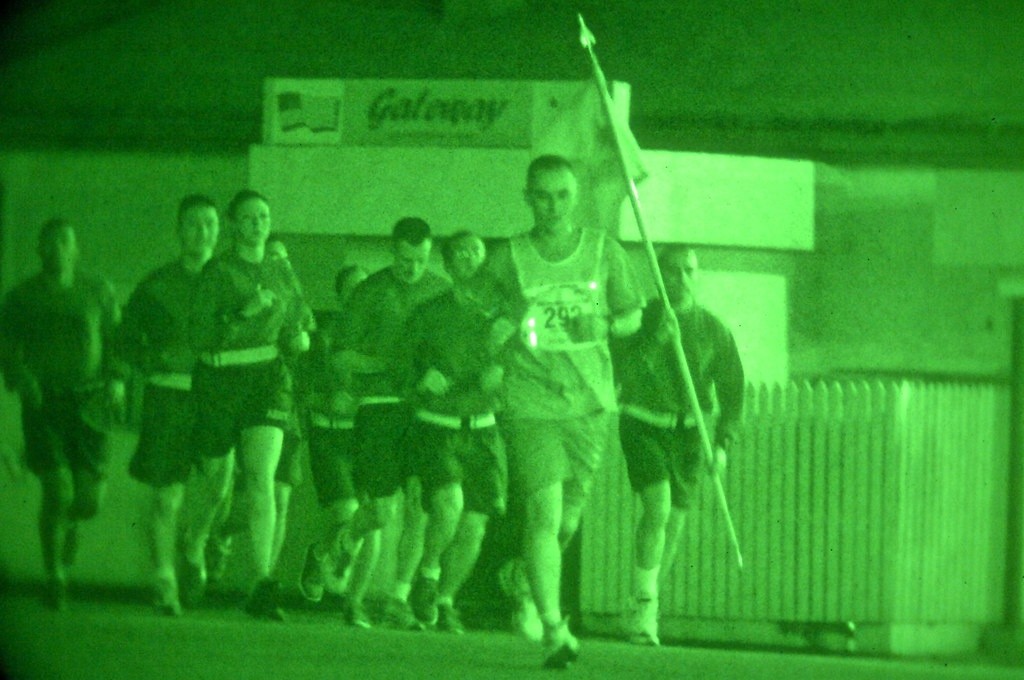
[61,515,79,564]
[245,576,279,614]
[139,568,177,608]
[206,532,234,581]
[46,573,65,608]
[177,529,206,603]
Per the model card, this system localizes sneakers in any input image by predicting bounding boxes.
[407,572,439,624]
[544,627,580,670]
[628,615,658,645]
[374,595,427,631]
[322,524,363,595]
[511,609,543,642]
[344,599,372,627]
[437,602,466,637]
[298,544,329,602]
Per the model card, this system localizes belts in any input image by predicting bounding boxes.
[148,371,191,392]
[309,410,357,432]
[357,396,401,405]
[622,403,695,431]
[418,408,496,430]
[200,345,281,367]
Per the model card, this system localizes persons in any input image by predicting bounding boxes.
[464,155,643,670]
[303,217,508,633]
[0,220,119,613]
[612,243,746,644]
[104,196,221,616]
[185,190,316,622]
